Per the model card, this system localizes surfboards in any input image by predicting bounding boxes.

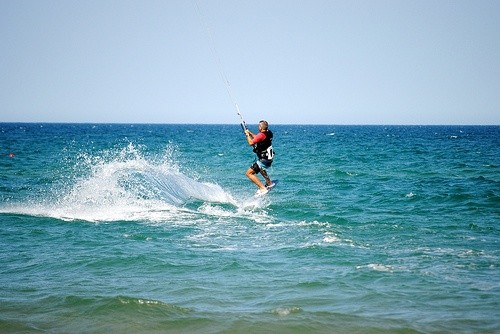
[252,181,276,202]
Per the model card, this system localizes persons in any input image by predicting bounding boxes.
[244,121,275,195]
